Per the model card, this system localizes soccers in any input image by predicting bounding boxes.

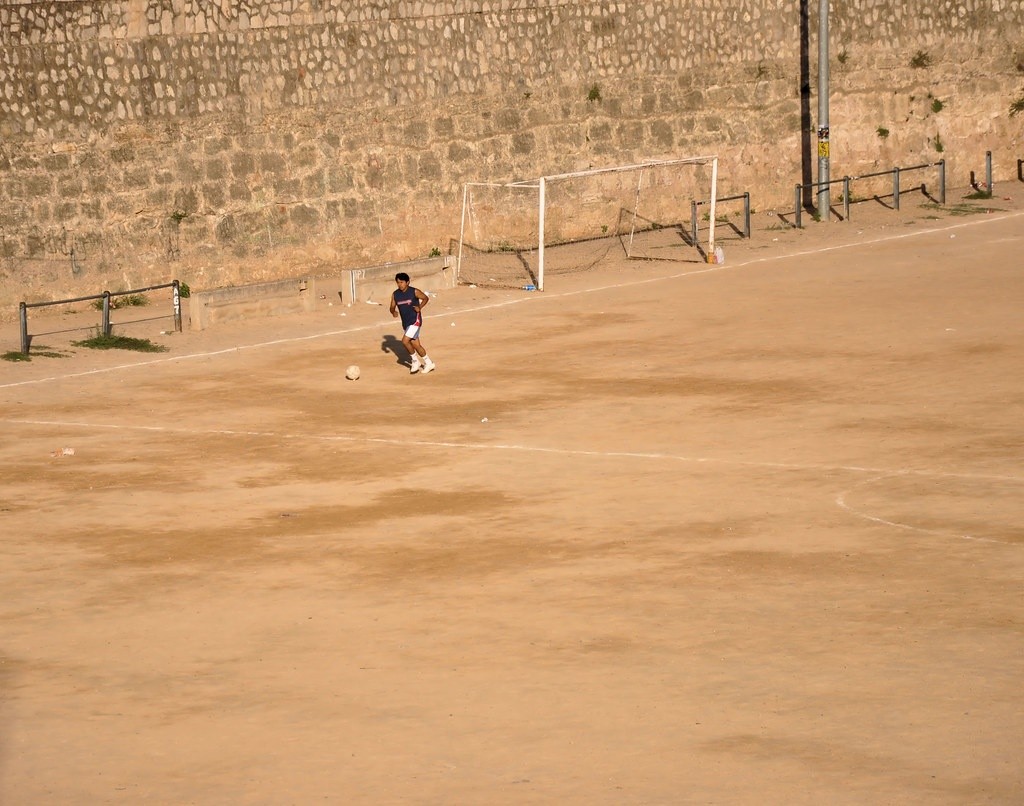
[345,365,361,380]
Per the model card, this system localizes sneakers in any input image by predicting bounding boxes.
[420,362,435,373]
[405,359,421,372]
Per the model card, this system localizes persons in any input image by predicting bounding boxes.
[390,273,435,373]
